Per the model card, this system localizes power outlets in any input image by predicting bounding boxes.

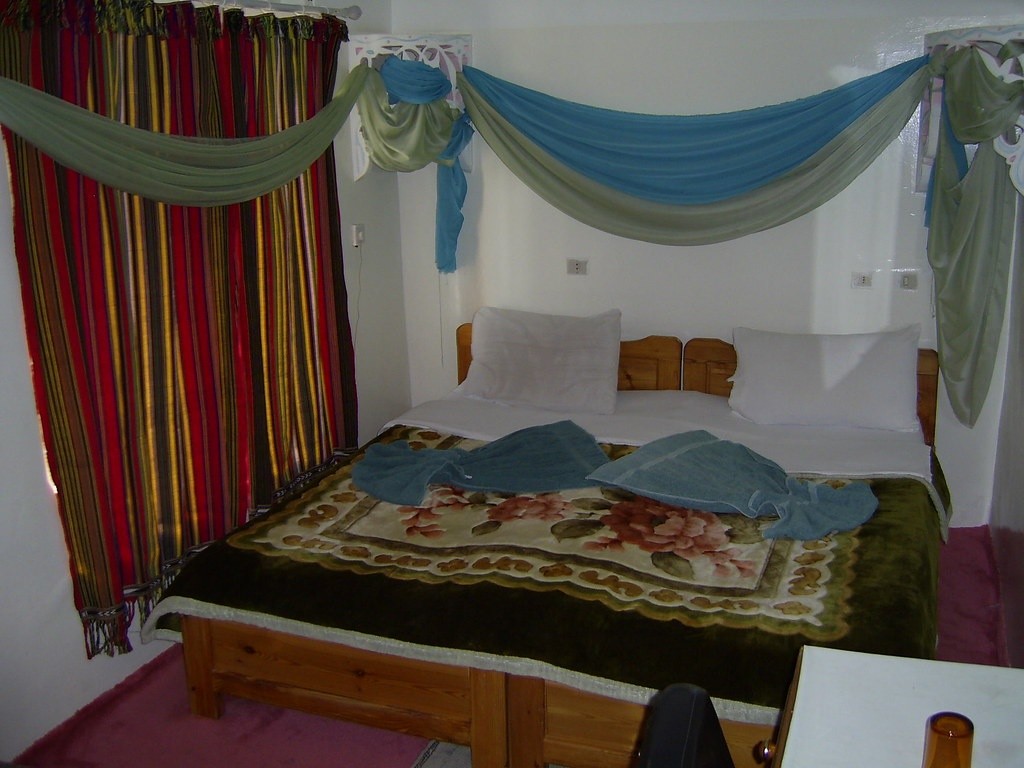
[351,223,366,247]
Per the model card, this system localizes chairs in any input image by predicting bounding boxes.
[620,683,733,767]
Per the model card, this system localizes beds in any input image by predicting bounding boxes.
[140,329,955,768]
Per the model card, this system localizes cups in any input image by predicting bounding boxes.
[921,712,974,768]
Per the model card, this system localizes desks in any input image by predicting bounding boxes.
[748,643,1024,768]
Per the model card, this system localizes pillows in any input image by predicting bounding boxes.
[444,306,623,417]
[730,320,924,432]
